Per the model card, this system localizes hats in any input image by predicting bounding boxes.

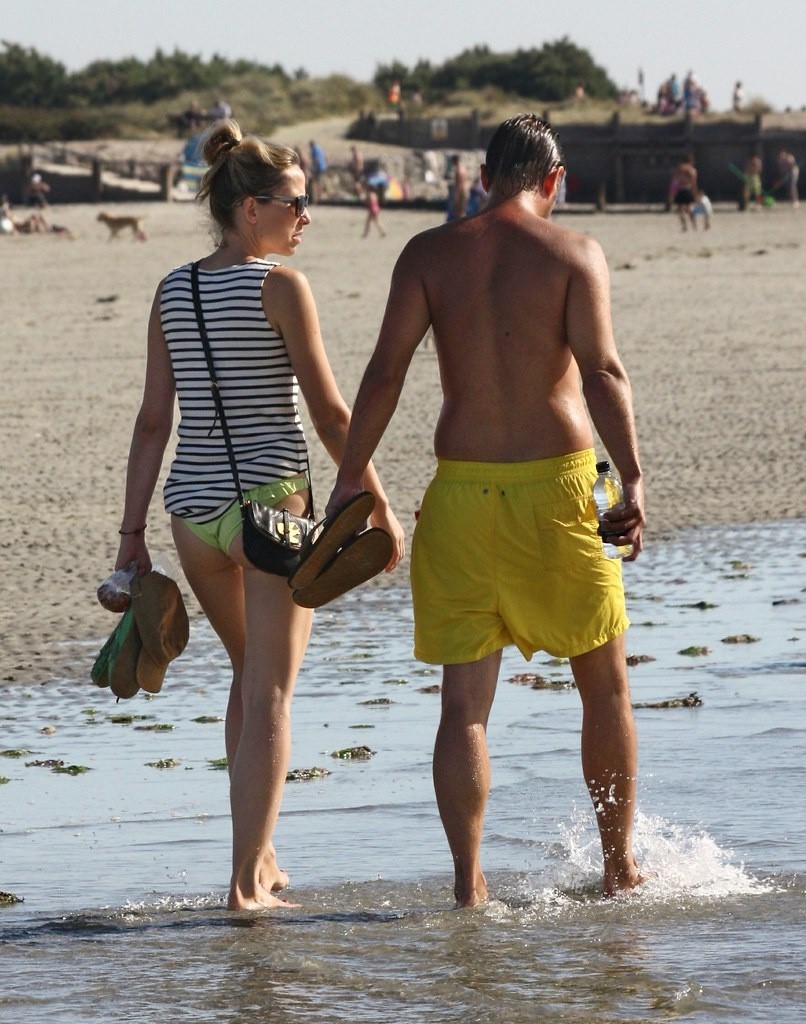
[129,571,189,693]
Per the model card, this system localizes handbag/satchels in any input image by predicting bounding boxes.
[238,500,319,577]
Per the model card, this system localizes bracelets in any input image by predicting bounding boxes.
[119,524,148,536]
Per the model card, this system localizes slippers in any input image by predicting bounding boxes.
[287,490,376,590]
[292,526,394,608]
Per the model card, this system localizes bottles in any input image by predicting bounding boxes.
[593,460,634,559]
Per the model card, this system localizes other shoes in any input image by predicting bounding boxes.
[91,610,128,688]
[109,604,142,699]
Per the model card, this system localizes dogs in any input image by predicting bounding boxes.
[98,212,145,239]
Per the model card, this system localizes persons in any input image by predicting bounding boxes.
[178,76,568,242]
[325,113,650,911]
[668,153,698,231]
[741,149,764,210]
[778,149,802,207]
[694,191,713,231]
[111,119,405,913]
[637,71,749,122]
[0,173,55,234]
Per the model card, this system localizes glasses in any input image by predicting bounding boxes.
[232,193,309,217]
